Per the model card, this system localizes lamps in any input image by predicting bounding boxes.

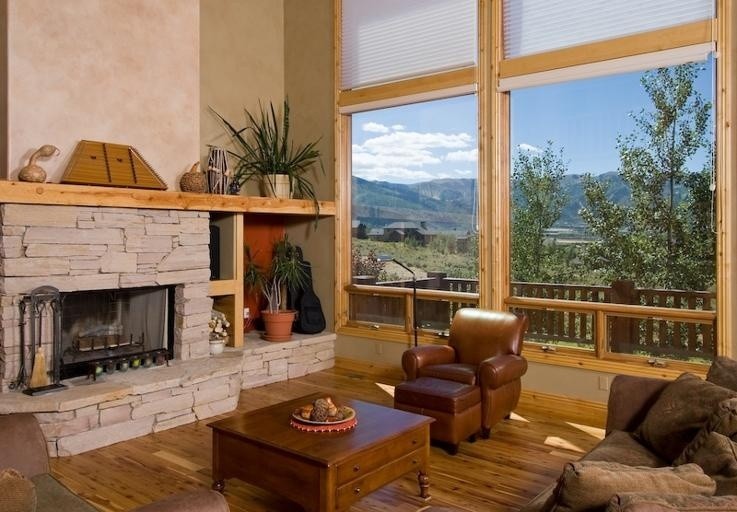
[375,255,419,347]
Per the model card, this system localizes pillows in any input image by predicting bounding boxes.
[0,467,38,512]
[632,371,737,461]
[671,397,737,467]
[604,492,737,512]
[539,459,719,512]
[706,356,737,392]
[692,431,737,476]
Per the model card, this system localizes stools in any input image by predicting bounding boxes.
[394,376,483,455]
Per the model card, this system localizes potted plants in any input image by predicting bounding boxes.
[204,92,328,231]
[241,232,314,343]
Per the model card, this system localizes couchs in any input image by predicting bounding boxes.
[520,374,737,512]
[0,412,231,512]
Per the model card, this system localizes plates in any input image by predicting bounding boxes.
[291,406,356,424]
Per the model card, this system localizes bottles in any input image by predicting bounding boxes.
[30,347,49,388]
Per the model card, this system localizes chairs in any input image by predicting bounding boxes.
[402,307,528,439]
